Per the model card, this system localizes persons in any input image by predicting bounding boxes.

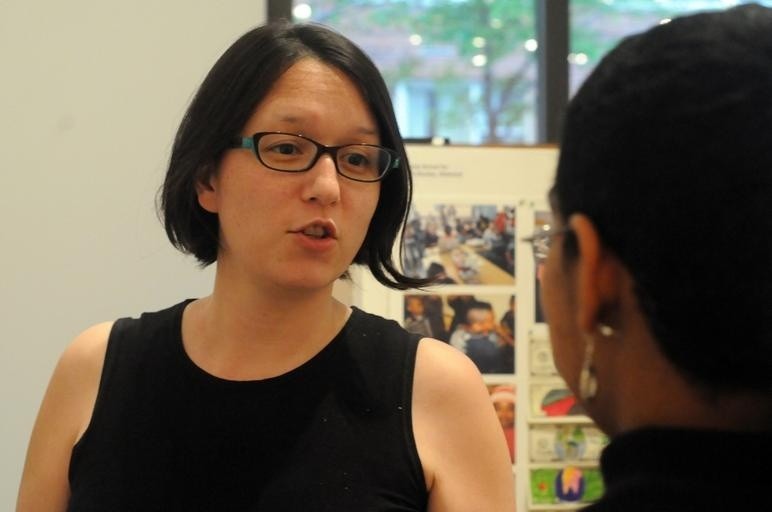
[541,4,772,512]
[390,200,515,462]
[16,21,515,512]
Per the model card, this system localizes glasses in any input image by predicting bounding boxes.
[225,132,401,182]
[521,226,572,264]
